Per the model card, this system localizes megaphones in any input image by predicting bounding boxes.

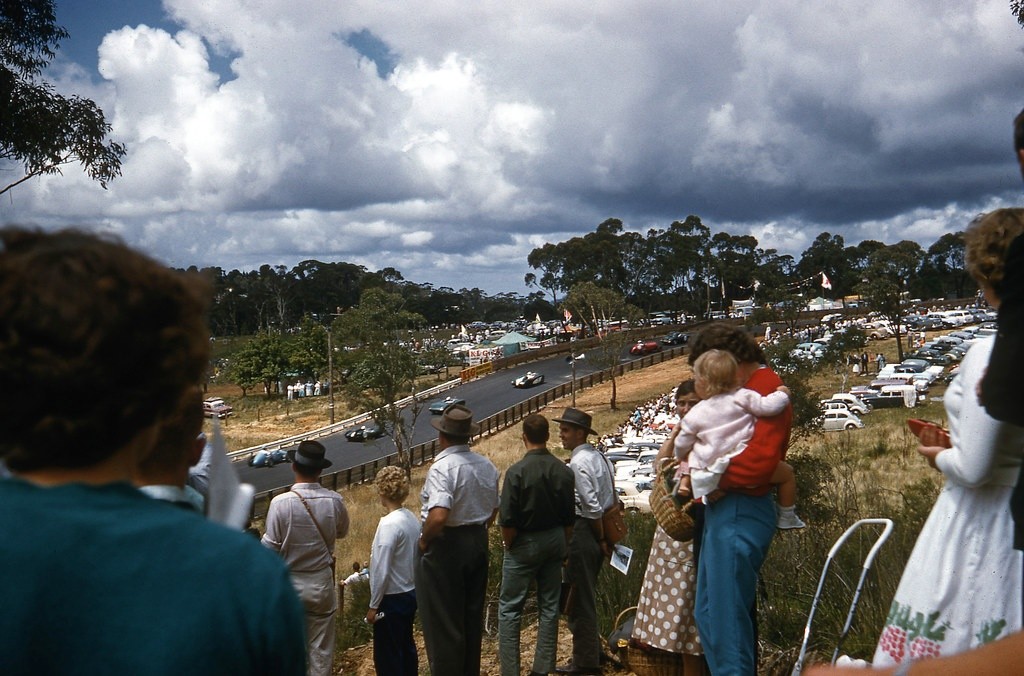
[575,353,585,360]
[566,356,575,364]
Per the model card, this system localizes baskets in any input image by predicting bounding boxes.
[649,457,700,542]
[615,607,682,676]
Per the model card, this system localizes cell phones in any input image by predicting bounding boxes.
[907,419,952,449]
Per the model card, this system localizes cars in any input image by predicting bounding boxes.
[203,398,233,419]
[401,318,627,370]
[760,303,997,433]
[603,401,682,514]
[637,306,755,324]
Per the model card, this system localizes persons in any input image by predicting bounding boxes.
[286,378,330,401]
[904,327,926,348]
[629,379,711,676]
[594,289,990,451]
[260,439,350,676]
[551,406,620,676]
[976,109,1024,552]
[0,228,310,676]
[852,350,885,377]
[597,393,671,455]
[804,630,1024,676]
[366,465,421,676]
[497,414,576,676]
[136,393,212,515]
[413,403,501,676]
[673,348,806,530]
[673,327,792,676]
[341,561,369,586]
[870,206,1024,676]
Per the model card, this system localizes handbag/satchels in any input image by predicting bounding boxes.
[603,505,627,544]
[330,555,336,588]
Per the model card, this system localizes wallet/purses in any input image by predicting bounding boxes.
[907,417,952,450]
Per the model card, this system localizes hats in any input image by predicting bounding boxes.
[431,404,481,436]
[552,408,598,435]
[287,440,332,469]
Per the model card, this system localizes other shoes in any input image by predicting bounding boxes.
[777,515,805,529]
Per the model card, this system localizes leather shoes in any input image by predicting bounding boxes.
[556,661,605,675]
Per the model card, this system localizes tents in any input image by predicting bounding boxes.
[490,331,536,345]
